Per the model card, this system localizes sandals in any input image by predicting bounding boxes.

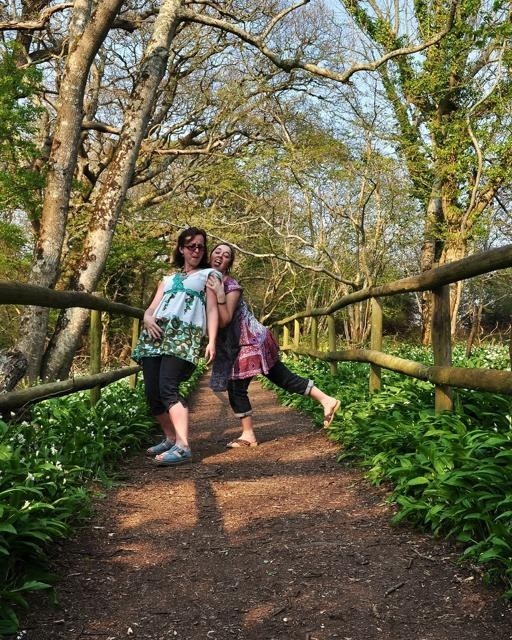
[142,436,176,456]
[152,444,195,468]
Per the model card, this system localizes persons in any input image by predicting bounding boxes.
[131,227,221,465]
[167,243,342,448]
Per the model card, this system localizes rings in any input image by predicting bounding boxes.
[211,282,214,286]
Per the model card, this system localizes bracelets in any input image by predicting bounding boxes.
[217,301,227,305]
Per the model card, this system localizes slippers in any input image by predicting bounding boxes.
[224,436,260,451]
[322,394,344,433]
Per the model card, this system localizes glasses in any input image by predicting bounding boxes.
[182,243,207,253]
